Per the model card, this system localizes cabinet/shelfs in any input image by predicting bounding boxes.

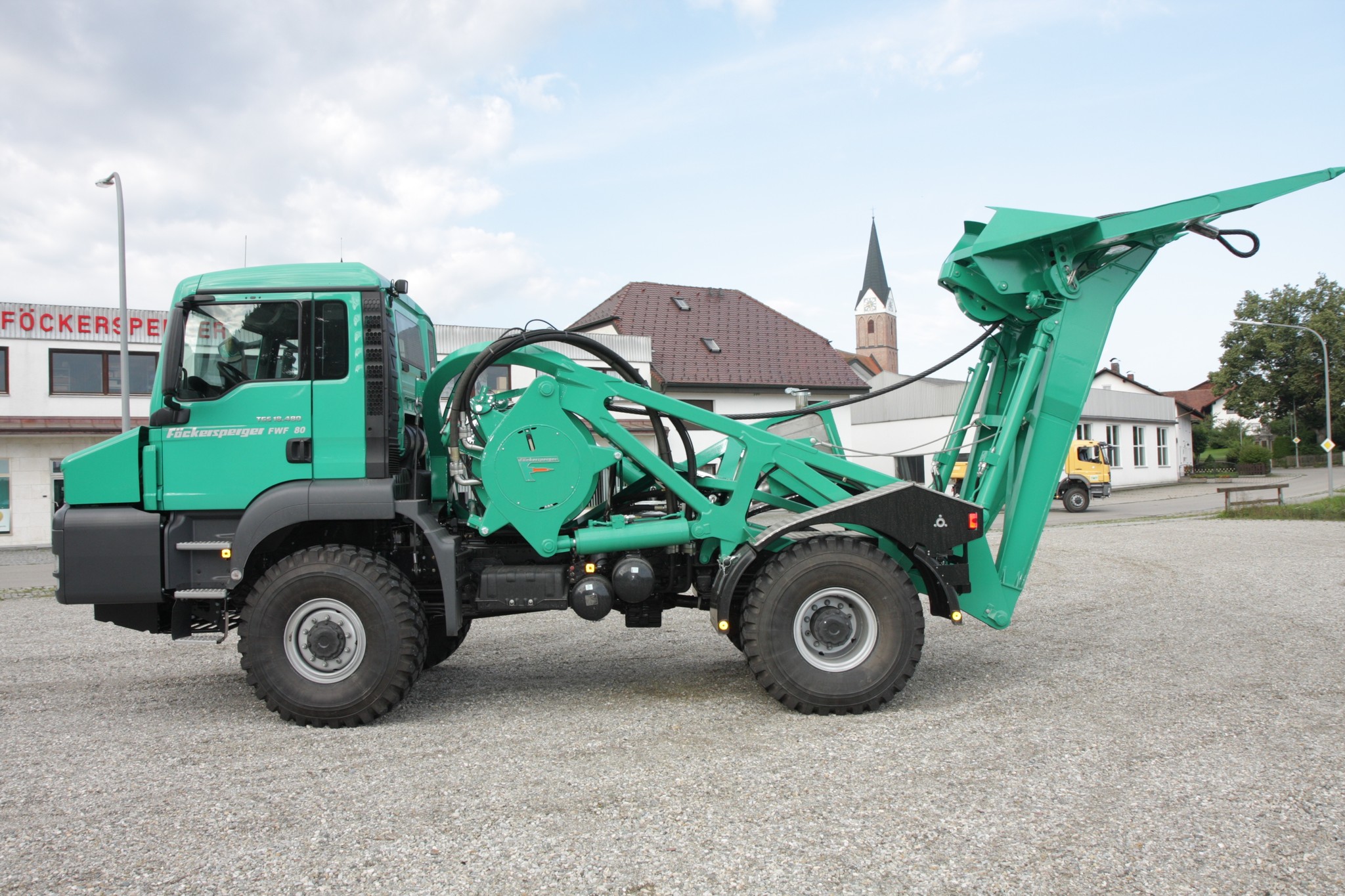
[100,363,121,393]
[53,364,70,392]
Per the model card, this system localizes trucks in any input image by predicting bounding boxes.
[949,439,1112,513]
[48,163,1345,730]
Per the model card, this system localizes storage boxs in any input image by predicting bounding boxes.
[109,370,120,387]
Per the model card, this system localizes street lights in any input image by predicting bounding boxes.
[95,171,131,435]
[1228,320,1334,500]
